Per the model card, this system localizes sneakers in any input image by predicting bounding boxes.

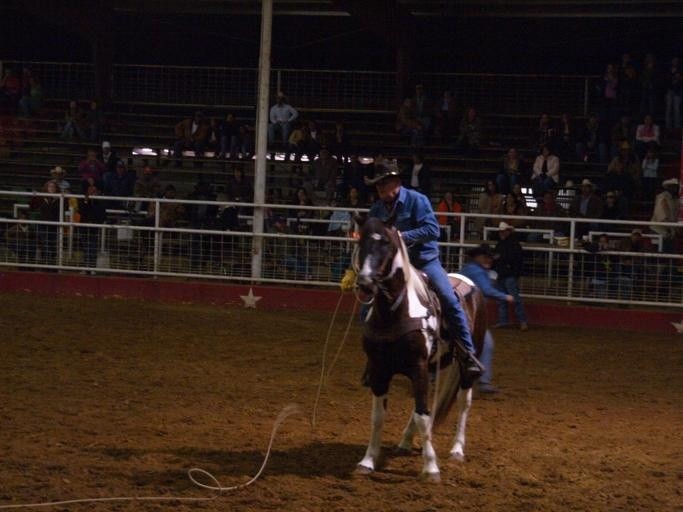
[488,321,529,332]
[451,352,482,390]
[478,384,501,394]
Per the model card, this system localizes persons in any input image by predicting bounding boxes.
[454,244,514,394]
[491,222,529,331]
[364,162,481,376]
[2,53,682,309]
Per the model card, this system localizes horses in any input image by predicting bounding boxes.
[347,200,487,485]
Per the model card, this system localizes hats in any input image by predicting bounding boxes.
[49,141,154,178]
[467,242,497,258]
[362,160,405,187]
[498,111,680,231]
